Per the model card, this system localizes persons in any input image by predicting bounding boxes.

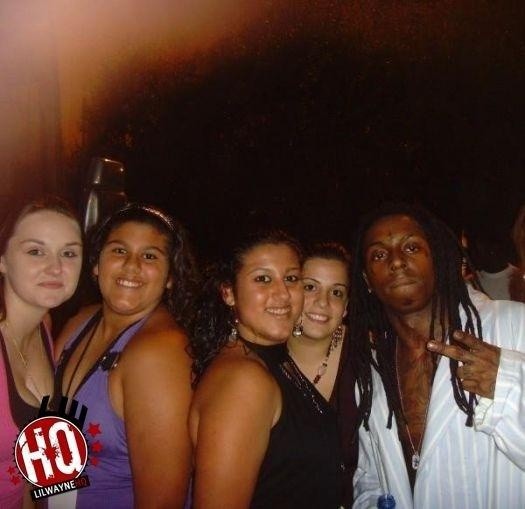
[183,203,523,509]
[0,201,84,509]
[54,205,192,508]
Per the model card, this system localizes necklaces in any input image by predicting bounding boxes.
[5,318,47,408]
[65,310,137,398]
